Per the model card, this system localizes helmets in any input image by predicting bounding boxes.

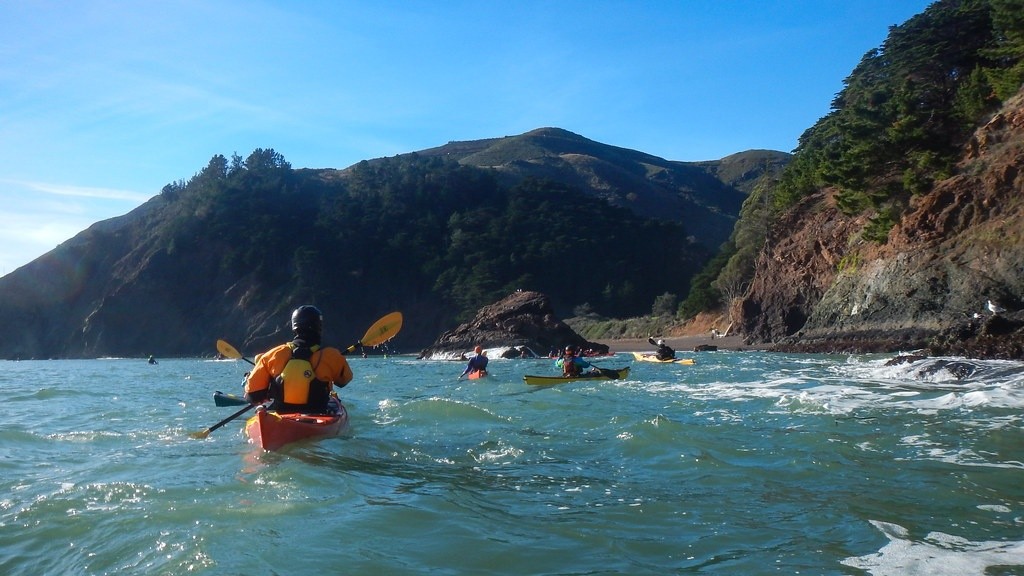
[658,340,666,345]
[475,346,482,354]
[290,304,323,333]
[565,344,574,351]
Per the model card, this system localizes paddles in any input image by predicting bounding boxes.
[647,338,661,348]
[214,337,260,368]
[457,350,488,381]
[585,361,622,382]
[188,309,405,444]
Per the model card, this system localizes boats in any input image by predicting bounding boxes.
[145,362,159,366]
[694,344,717,351]
[363,355,367,358]
[631,352,696,365]
[515,352,614,359]
[244,389,348,452]
[469,369,487,381]
[384,356,388,359]
[524,365,631,385]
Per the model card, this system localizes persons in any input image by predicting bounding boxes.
[655,339,675,360]
[148,355,159,365]
[241,305,353,415]
[462,346,489,375]
[548,346,592,378]
